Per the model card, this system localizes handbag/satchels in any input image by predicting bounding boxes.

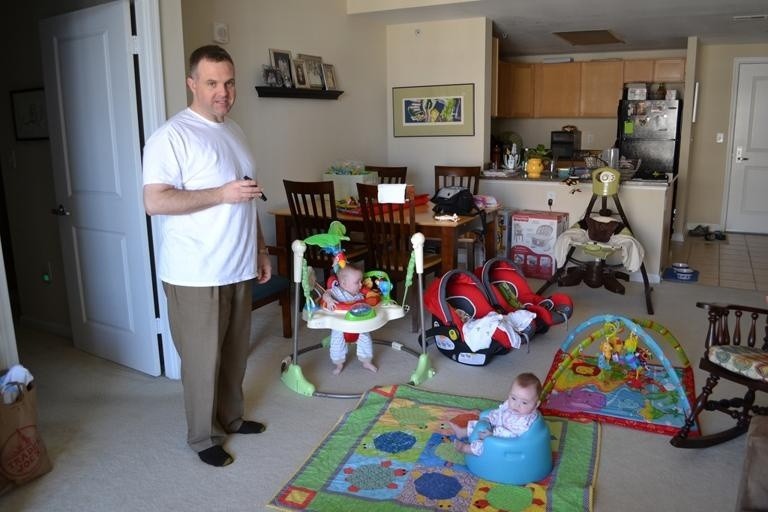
[0,370,52,496]
[426,186,474,216]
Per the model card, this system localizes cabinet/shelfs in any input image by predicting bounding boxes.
[497,58,685,119]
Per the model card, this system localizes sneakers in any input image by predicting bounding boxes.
[688,224,707,236]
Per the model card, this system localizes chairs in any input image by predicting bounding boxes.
[282,178,367,311]
[434,165,481,277]
[364,165,408,246]
[669,301,768,448]
[356,182,441,334]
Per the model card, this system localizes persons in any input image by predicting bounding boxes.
[142,44,272,468]
[297,64,305,85]
[322,264,377,376]
[447,372,542,456]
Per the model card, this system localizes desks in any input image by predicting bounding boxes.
[267,200,480,338]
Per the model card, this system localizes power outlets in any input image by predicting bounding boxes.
[213,21,229,42]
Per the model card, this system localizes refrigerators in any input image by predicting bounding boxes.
[617,97,680,182]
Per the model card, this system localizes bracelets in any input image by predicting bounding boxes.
[259,247,269,254]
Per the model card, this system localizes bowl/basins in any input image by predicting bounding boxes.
[676,268,694,281]
[557,167,570,180]
[671,262,689,276]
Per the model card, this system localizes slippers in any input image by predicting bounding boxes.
[704,233,715,241]
[714,231,725,241]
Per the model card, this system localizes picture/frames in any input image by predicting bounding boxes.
[392,83,475,136]
[269,48,337,91]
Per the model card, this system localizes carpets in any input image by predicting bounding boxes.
[266,384,601,512]
